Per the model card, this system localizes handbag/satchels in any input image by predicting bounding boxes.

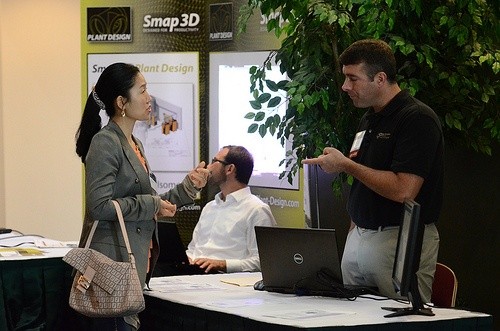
[61,199,146,319]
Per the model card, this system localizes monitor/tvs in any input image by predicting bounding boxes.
[381,198,435,318]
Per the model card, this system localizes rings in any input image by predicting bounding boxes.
[203,177,206,181]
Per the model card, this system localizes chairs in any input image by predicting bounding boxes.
[431,263,458,307]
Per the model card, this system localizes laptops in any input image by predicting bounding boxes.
[158,220,198,267]
[254,226,376,298]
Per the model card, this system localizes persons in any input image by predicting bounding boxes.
[70,62,210,331]
[301,40,444,304]
[185,145,277,274]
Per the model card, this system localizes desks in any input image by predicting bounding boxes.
[143,271,493,331]
[0,233,79,331]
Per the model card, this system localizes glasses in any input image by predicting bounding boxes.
[212,159,228,166]
